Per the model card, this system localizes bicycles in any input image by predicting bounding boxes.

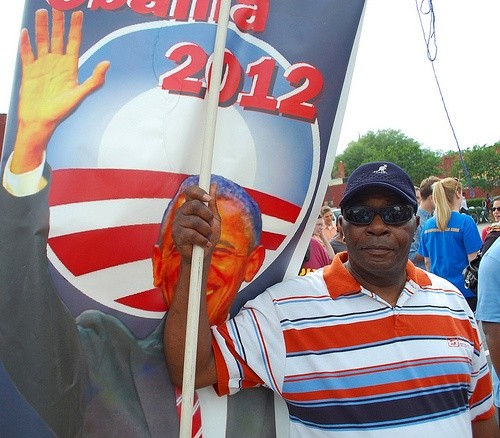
[470,205,493,225]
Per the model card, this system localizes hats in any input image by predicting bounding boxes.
[339,162,419,215]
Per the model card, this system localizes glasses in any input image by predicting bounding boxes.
[492,207,500,212]
[342,202,414,225]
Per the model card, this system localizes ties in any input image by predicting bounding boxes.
[172,388,203,438]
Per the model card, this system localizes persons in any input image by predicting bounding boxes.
[475,235,500,423]
[0,7,277,438]
[166,162,500,438]
[298,176,500,313]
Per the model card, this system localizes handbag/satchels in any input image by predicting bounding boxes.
[463,234,499,295]
